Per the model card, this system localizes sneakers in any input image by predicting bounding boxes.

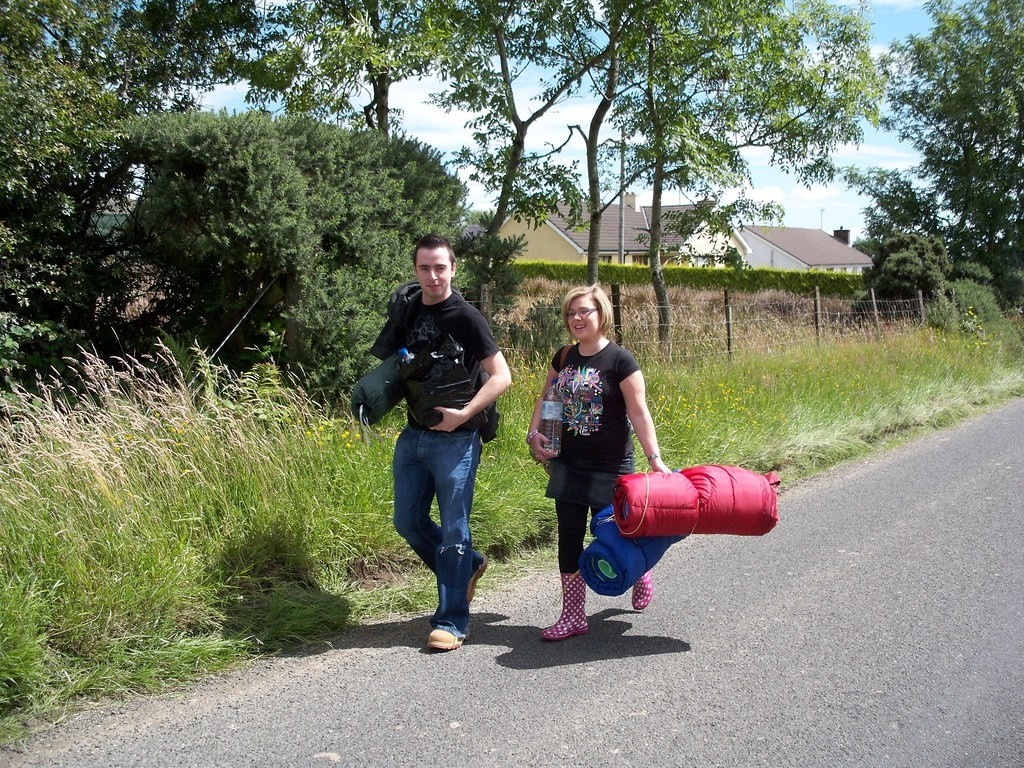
[467,555,488,603]
[427,629,464,649]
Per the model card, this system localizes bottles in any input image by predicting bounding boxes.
[399,347,416,364]
[538,378,564,457]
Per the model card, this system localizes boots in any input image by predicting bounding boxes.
[632,568,653,610]
[540,572,589,641]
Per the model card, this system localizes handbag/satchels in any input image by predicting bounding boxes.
[397,332,488,432]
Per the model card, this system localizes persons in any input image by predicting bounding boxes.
[525,286,675,643]
[381,234,513,652]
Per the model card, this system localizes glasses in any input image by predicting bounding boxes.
[564,307,599,319]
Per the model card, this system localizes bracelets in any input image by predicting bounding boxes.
[647,453,661,462]
[525,429,538,443]
